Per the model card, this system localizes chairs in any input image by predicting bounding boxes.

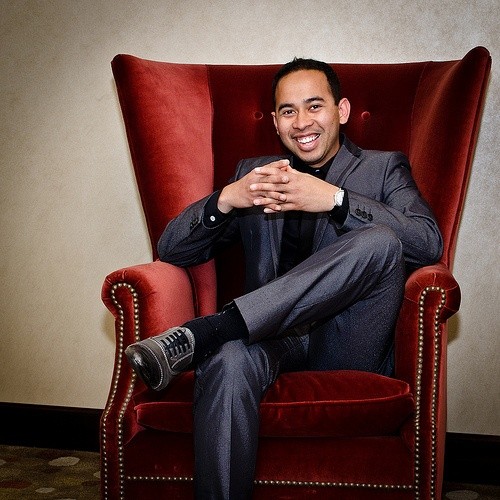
[99,46,495,500]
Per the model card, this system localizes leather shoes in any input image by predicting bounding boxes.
[125,327,196,390]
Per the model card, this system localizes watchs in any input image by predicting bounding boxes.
[334,187,344,206]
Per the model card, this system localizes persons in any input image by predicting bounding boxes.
[126,58,444,500]
